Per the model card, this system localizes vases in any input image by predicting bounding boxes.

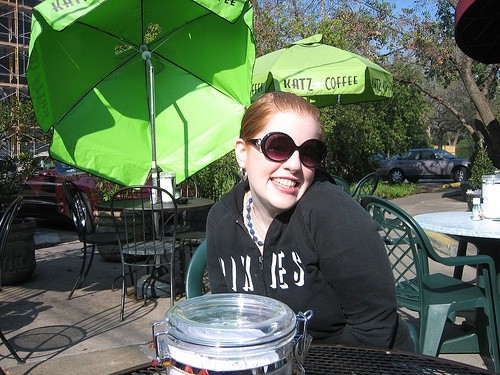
[466,195,483,211]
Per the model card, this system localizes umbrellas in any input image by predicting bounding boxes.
[26,0,256,266]
[250,33,393,108]
[454,0,500,64]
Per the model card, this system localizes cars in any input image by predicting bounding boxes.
[9,148,151,234]
[374,147,473,185]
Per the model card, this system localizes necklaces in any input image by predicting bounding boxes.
[245,198,264,247]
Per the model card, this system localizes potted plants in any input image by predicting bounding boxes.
[471,139,500,219]
[71,172,151,262]
[0,152,36,284]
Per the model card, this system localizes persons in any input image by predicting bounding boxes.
[206,90,397,351]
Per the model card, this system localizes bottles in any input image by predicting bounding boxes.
[472,198,481,220]
[480,174,500,221]
[144,292,314,375]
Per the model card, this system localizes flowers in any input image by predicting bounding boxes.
[466,189,482,195]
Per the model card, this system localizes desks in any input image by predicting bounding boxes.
[413,212,500,280]
[108,346,500,375]
[94,197,216,289]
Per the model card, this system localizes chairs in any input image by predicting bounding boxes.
[331,172,500,373]
[0,196,25,375]
[61,179,206,321]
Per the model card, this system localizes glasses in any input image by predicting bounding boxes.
[244,131,329,170]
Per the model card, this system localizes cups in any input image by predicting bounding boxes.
[159,171,176,203]
